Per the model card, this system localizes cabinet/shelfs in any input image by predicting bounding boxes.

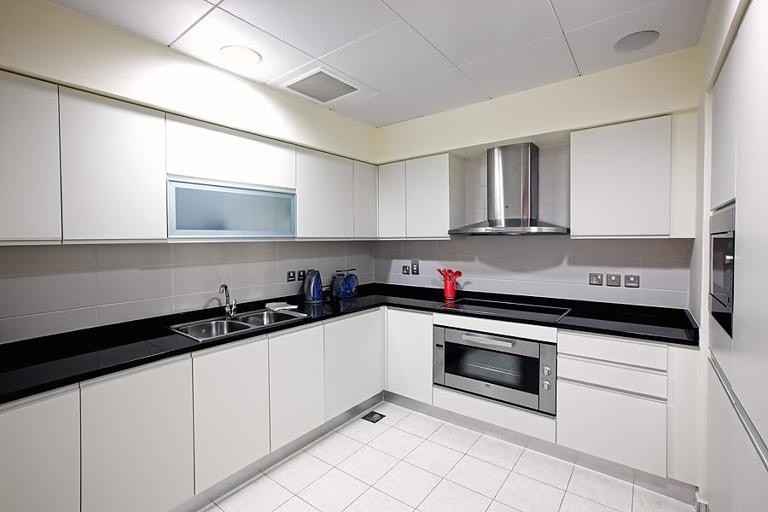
[706,42,737,215]
[58,84,167,244]
[405,150,466,242]
[297,146,352,241]
[555,329,768,512]
[352,160,406,241]
[570,113,672,239]
[167,112,298,195]
[1,69,62,248]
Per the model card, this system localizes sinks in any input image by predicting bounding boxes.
[234,307,308,327]
[168,318,251,343]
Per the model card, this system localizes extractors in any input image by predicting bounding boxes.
[447,141,570,237]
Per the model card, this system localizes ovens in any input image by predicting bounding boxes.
[431,324,559,422]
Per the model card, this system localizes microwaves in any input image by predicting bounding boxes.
[706,209,733,340]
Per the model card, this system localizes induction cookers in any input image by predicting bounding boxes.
[435,297,573,323]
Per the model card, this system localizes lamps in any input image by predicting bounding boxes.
[614,31,660,53]
[219,46,262,66]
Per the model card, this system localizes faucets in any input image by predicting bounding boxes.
[219,283,231,316]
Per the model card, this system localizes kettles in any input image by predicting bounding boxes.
[303,269,323,304]
